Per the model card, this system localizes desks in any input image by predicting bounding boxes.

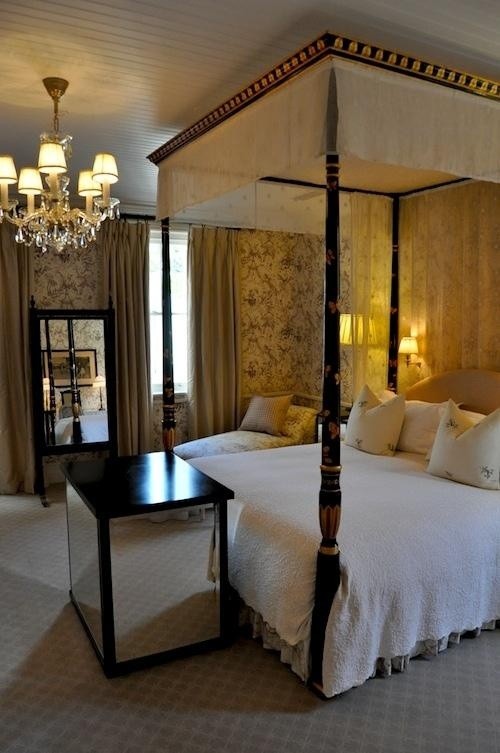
[59,449,235,680]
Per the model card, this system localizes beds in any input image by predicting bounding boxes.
[172,404,319,460]
[54,389,109,446]
[146,35,500,700]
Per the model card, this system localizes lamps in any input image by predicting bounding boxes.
[339,312,376,411]
[398,337,419,367]
[0,77,121,255]
[93,374,107,411]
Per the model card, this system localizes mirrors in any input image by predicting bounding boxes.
[28,294,121,508]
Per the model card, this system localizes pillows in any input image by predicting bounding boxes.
[343,383,405,456]
[378,390,460,456]
[79,387,101,411]
[437,401,487,438]
[238,394,293,437]
[424,399,500,491]
[60,406,84,418]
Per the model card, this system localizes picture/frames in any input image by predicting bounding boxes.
[41,349,98,388]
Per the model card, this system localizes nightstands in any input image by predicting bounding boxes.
[315,410,350,443]
